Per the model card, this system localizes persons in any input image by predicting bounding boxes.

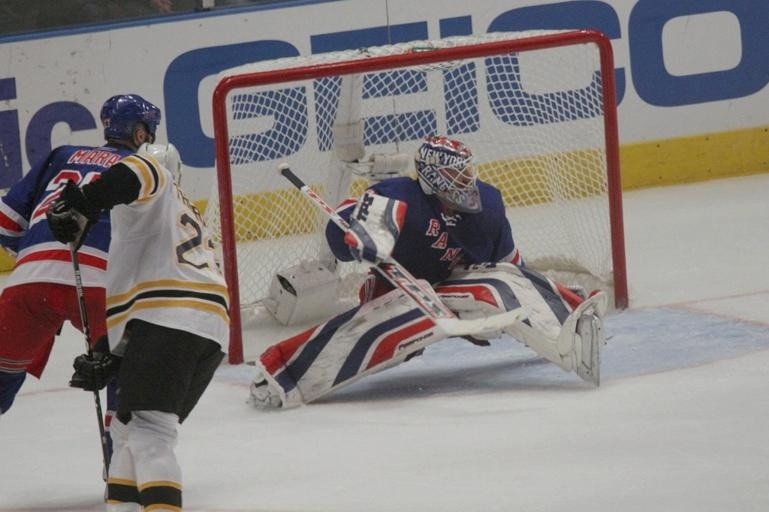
[44,140,233,512]
[0,93,162,480]
[251,136,607,412]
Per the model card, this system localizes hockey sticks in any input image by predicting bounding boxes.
[278,163,532,335]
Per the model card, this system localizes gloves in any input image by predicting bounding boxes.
[45,178,94,251]
[68,351,118,391]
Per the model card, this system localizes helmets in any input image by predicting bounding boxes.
[414,134,483,214]
[99,94,182,187]
